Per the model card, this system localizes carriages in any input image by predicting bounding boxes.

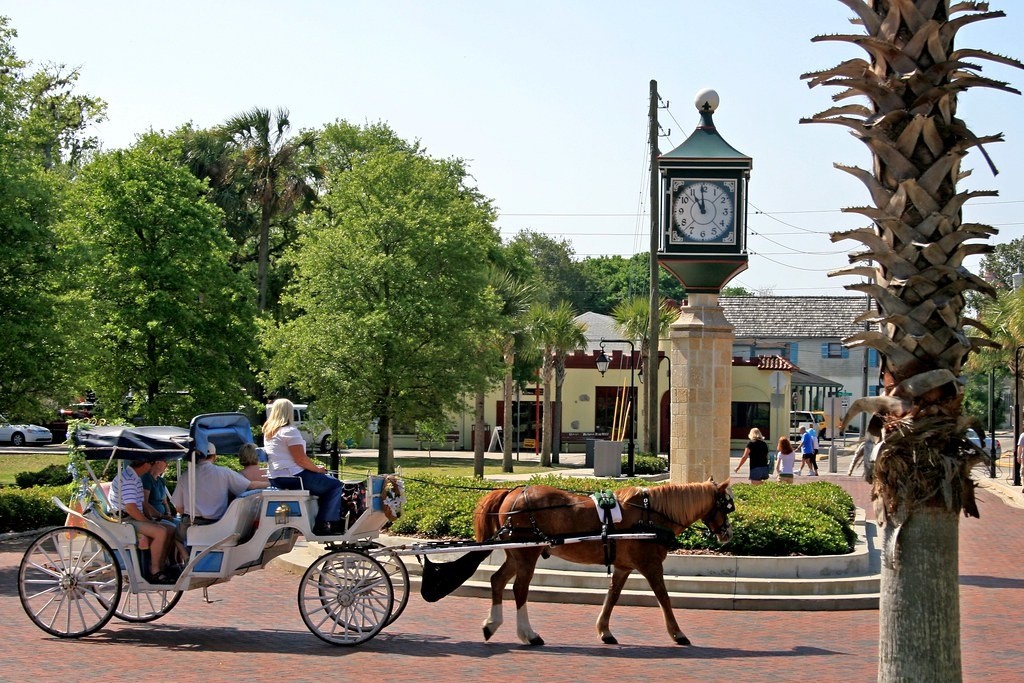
[16,414,746,652]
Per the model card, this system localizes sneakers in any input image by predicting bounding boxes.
[793,470,801,476]
[806,471,815,476]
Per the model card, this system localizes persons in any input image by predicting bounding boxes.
[776,437,795,483]
[735,428,769,482]
[1016,433,1024,493]
[795,426,817,475]
[263,399,344,536]
[107,460,171,582]
[170,442,271,564]
[141,461,190,563]
[805,424,818,476]
[237,443,269,482]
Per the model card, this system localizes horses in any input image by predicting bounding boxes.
[474,472,733,647]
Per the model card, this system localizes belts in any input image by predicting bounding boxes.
[182,514,218,522]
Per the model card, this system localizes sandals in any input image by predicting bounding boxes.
[145,571,176,584]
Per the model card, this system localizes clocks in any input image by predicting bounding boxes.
[669,178,738,245]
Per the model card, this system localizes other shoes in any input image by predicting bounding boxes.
[312,521,344,535]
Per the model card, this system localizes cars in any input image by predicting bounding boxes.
[959,428,1002,466]
[0,411,52,445]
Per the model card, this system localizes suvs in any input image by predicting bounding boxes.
[789,410,820,442]
[59,402,100,418]
[810,411,843,441]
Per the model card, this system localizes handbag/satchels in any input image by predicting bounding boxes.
[764,441,775,474]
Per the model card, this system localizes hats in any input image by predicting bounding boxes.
[196,442,216,455]
[808,428,816,436]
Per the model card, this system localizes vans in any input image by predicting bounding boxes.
[265,404,334,453]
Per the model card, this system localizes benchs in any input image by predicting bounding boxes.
[86,482,180,571]
[186,488,266,547]
[262,488,319,535]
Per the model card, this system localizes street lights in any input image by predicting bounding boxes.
[791,391,799,441]
[596,336,643,476]
[637,353,672,472]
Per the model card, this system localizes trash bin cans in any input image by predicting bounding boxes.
[584,439,594,467]
